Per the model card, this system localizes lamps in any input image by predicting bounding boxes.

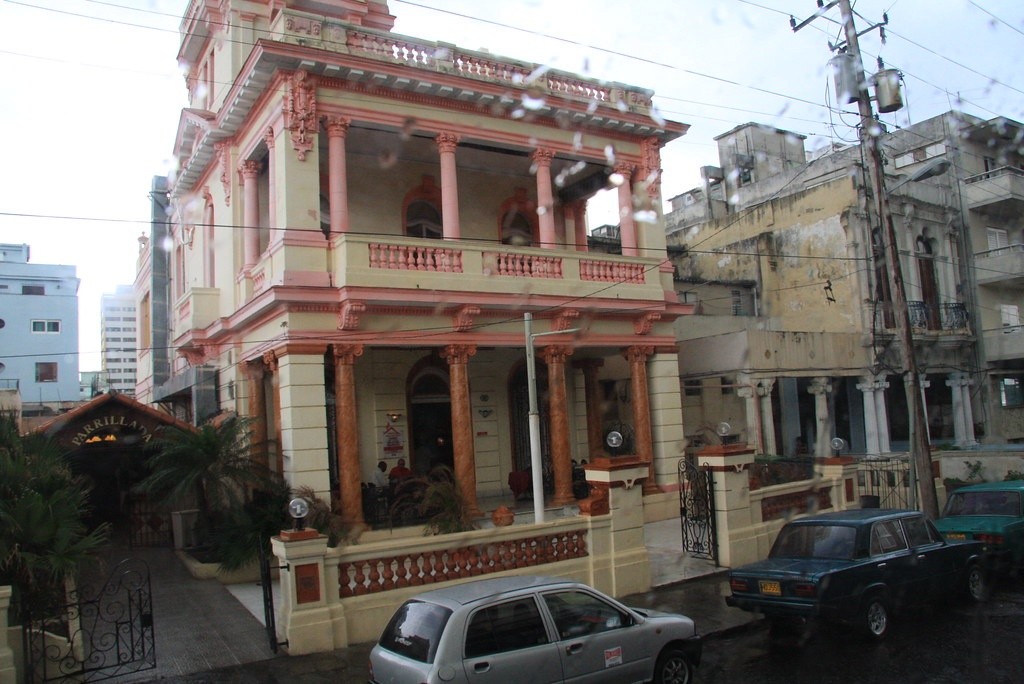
[830,437,844,457]
[479,410,493,418]
[387,413,401,422]
[288,498,309,531]
[607,431,622,456]
[716,422,730,445]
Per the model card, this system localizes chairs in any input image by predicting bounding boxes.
[361,482,387,514]
[508,471,533,500]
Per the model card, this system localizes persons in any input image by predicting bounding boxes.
[389,459,412,481]
[371,461,390,488]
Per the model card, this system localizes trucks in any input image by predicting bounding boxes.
[368,574,702,684]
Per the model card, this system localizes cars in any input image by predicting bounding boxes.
[725,507,996,645]
[917,480,1024,579]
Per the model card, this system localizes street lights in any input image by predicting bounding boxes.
[826,40,938,526]
